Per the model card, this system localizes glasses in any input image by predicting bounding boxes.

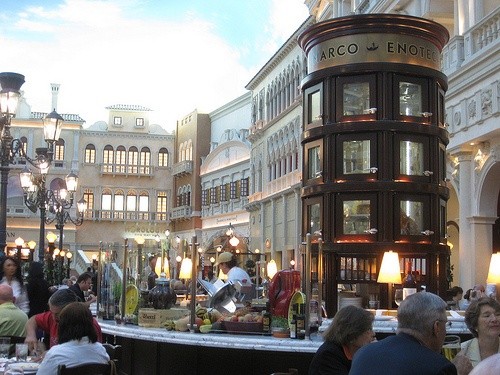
[435,319,451,329]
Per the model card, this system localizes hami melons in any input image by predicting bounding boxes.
[211,309,221,319]
[173,316,190,331]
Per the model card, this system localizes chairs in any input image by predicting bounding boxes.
[58,359,118,375]
[0,336,26,356]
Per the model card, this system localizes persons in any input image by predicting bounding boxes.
[213,252,251,285]
[207,271,217,283]
[307,286,500,375]
[0,256,110,375]
[140,256,157,289]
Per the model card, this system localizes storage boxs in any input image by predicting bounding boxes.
[138,308,190,328]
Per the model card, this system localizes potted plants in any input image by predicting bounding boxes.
[403,273,417,301]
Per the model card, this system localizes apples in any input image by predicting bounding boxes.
[195,318,202,325]
[203,319,211,325]
[231,314,263,323]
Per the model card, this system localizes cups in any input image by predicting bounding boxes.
[0,337,10,368]
[138,307,155,326]
[156,310,172,324]
[442,336,462,361]
[16,344,28,362]
[10,367,24,375]
[170,309,188,331]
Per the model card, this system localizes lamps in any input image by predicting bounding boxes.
[377,250,403,310]
[364,228,378,235]
[421,112,432,118]
[366,167,378,173]
[422,230,435,236]
[486,251,500,304]
[179,258,192,299]
[267,262,277,281]
[154,256,170,278]
[367,108,377,114]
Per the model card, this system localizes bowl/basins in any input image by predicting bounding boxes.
[224,321,263,331]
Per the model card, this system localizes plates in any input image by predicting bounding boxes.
[8,363,40,372]
[119,285,139,314]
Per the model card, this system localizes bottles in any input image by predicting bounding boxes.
[290,303,305,339]
[263,302,272,332]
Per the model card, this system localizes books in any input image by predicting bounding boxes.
[197,279,225,297]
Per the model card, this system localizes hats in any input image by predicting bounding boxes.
[213,252,234,267]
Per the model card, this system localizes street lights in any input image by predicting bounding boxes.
[229,235,240,267]
[10,108,88,286]
[0,71,23,257]
[134,235,146,274]
[153,227,180,261]
[210,256,216,278]
